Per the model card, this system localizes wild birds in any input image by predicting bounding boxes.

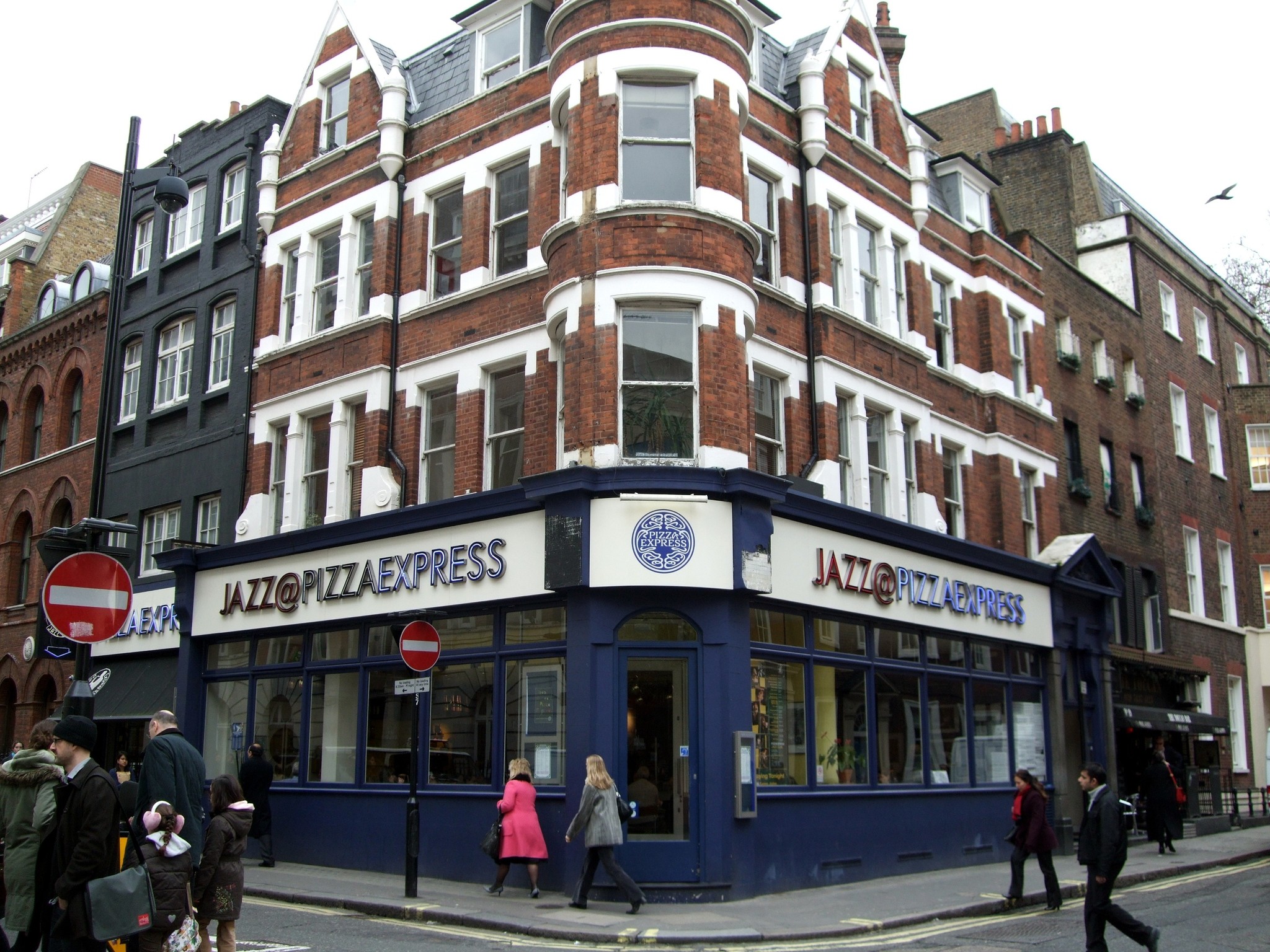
[1205,183,1237,204]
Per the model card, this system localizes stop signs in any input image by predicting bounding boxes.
[40,550,134,645]
[399,619,441,673]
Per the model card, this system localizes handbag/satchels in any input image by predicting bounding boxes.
[1004,818,1023,846]
[84,864,157,941]
[613,783,634,823]
[1176,786,1186,803]
[481,805,504,859]
[161,915,202,952]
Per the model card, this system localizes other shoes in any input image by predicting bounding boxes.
[1144,926,1160,952]
[258,862,275,867]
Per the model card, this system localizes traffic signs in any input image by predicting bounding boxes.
[394,676,430,695]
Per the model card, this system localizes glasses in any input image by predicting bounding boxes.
[146,724,154,738]
[52,738,63,745]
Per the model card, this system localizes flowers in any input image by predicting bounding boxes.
[819,731,867,774]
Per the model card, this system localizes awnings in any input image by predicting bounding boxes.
[1112,704,1231,736]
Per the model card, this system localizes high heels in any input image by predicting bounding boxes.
[483,886,503,896]
[1001,894,1023,903]
[626,897,647,914]
[1165,837,1176,853]
[1044,903,1059,910]
[1159,842,1165,854]
[569,901,587,909]
[528,888,539,899]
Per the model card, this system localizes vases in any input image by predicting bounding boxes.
[837,770,853,784]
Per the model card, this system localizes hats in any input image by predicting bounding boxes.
[53,715,97,752]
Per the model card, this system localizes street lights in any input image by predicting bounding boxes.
[55,115,190,728]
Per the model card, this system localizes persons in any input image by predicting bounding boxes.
[1140,732,1184,854]
[255,737,312,783]
[0,710,255,952]
[483,758,549,898]
[566,754,647,915]
[366,753,438,786]
[877,740,951,784]
[1002,770,1063,911]
[1078,762,1160,952]
[238,744,275,868]
[627,764,674,833]
